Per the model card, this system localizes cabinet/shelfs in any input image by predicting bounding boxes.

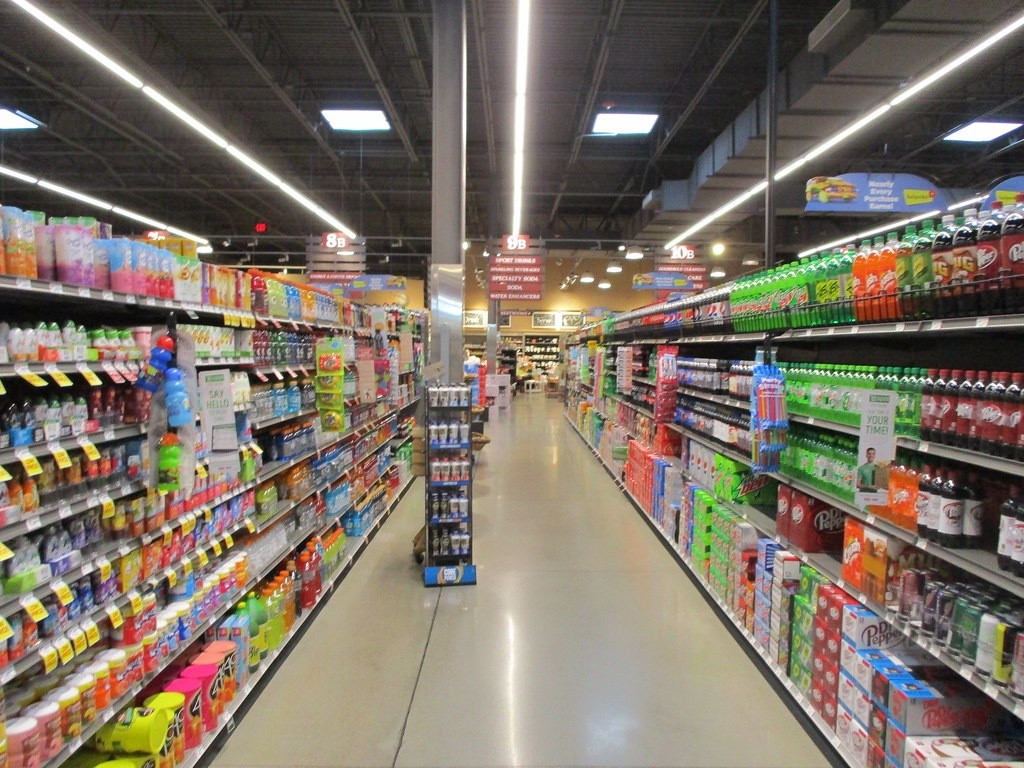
[500,195,1024,768]
[1,206,486,767]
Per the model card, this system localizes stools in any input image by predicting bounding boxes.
[524,380,542,394]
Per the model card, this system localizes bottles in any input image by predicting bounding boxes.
[234,269,421,671]
[575,193,1024,579]
[428,382,470,554]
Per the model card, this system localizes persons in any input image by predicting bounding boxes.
[857,447,881,492]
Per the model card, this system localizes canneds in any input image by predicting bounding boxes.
[899,568,1024,701]
[397,374,416,407]
